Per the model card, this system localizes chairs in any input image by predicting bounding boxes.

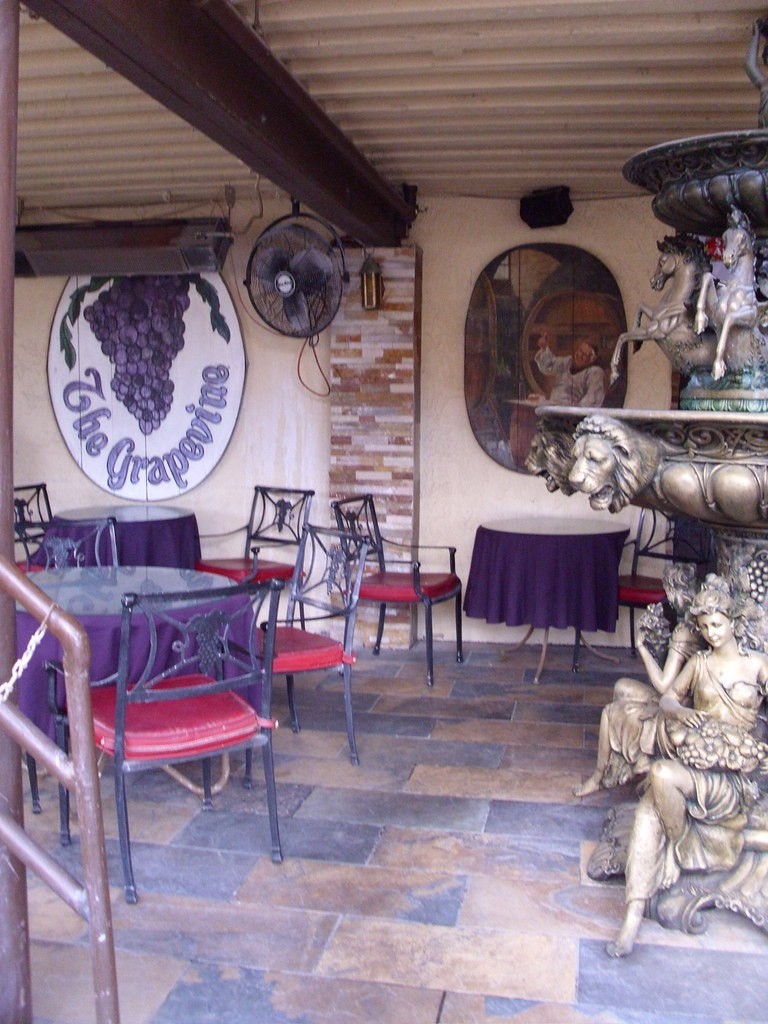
[195,485,315,630]
[14,482,53,572]
[572,507,708,674]
[41,577,286,906]
[14,516,121,572]
[217,523,370,789]
[330,493,463,687]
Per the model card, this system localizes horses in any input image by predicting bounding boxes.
[693,204,768,381]
[609,232,711,385]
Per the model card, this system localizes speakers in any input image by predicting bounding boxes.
[520,185,574,229]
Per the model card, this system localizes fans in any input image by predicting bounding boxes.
[243,197,351,339]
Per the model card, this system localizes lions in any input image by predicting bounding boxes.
[523,416,582,496]
[568,413,662,514]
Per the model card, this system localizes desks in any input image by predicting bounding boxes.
[15,567,262,753]
[30,504,202,570]
[462,516,631,684]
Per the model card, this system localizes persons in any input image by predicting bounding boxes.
[534,331,605,407]
[571,561,768,960]
[744,18,768,129]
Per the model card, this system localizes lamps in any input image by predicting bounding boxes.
[358,245,383,310]
